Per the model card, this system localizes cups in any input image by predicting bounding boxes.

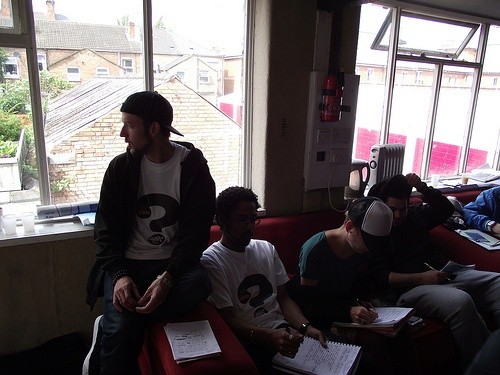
[1,214,17,235]
[20,211,35,232]
[431,174,440,187]
[461,172,468,184]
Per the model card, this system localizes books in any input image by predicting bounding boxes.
[341,306,418,336]
[272,336,362,375]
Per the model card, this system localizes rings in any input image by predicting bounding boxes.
[289,335,293,341]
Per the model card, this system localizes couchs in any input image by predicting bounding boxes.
[134,190,500,375]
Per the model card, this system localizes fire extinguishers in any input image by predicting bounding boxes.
[321,74,343,122]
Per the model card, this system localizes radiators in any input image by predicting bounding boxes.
[369,143,406,190]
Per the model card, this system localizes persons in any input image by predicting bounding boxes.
[200,188,329,375]
[299,172,500,375]
[81,91,217,375]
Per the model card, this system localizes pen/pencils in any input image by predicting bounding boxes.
[284,323,292,335]
[423,261,437,270]
[357,298,373,312]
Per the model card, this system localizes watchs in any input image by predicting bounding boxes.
[487,221,498,233]
[301,321,312,335]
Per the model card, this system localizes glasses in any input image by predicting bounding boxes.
[227,216,261,226]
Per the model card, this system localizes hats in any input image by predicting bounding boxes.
[347,196,395,257]
[120,90,184,137]
[366,173,412,197]
